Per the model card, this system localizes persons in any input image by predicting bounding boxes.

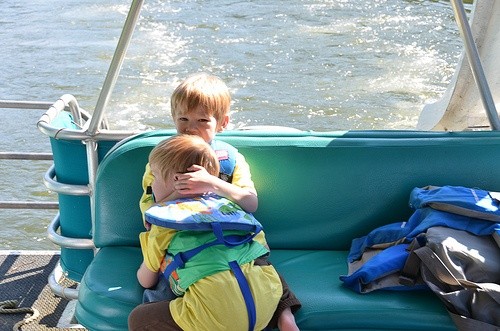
[126,133,301,331]
[139,73,259,279]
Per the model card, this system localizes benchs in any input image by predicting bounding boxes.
[73,132,499,330]
